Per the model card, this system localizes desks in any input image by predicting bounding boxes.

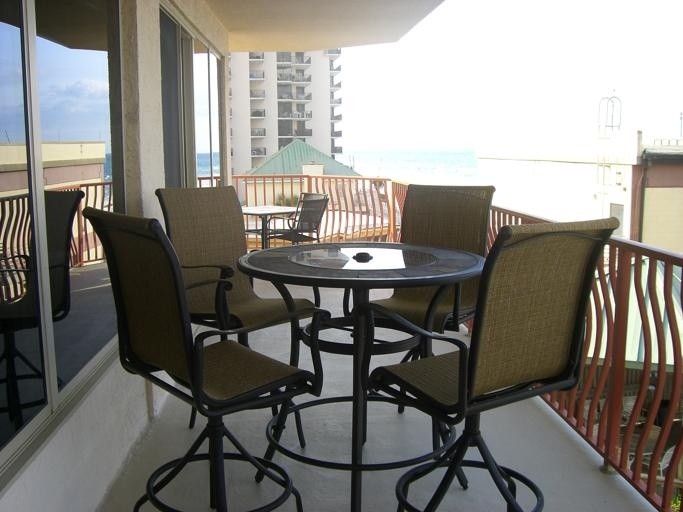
[241,205,318,250]
[236,243,488,512]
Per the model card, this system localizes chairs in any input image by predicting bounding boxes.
[342,184,495,412]
[0,190,85,421]
[154,186,319,428]
[369,218,619,512]
[268,193,329,245]
[82,207,331,512]
